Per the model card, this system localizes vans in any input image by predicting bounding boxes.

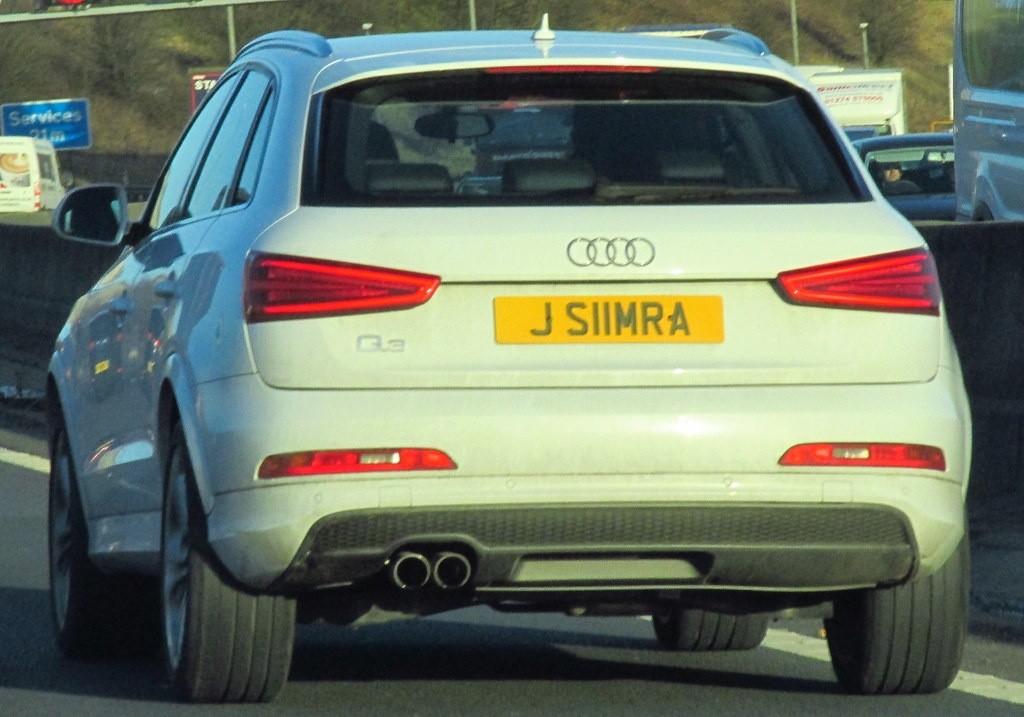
[0,136,67,214]
[951,0,1022,223]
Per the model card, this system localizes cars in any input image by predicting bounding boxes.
[846,125,958,221]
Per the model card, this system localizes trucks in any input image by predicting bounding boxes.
[793,62,907,148]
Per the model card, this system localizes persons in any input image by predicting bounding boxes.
[882,162,902,187]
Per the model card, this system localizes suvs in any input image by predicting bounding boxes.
[40,30,975,703]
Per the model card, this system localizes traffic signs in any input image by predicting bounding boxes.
[1,98,92,152]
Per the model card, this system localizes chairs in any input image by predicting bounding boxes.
[885,178,924,196]
[363,148,729,200]
